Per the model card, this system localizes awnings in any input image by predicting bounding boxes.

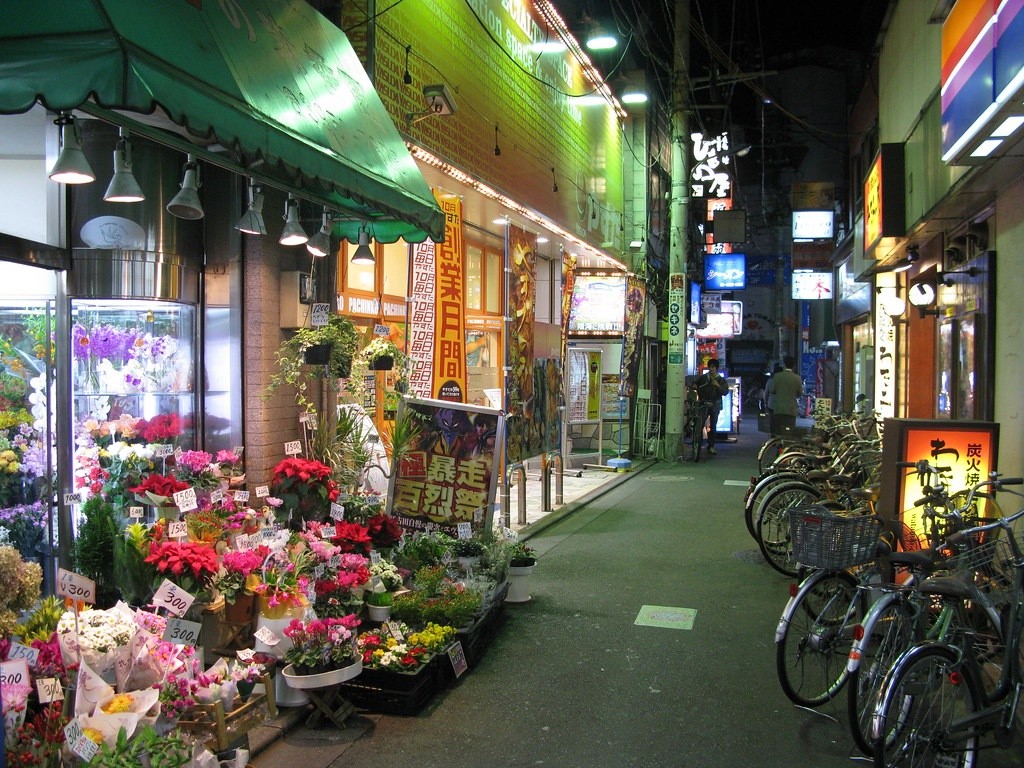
[0,0,446,244]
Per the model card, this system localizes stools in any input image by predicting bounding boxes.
[301,686,358,729]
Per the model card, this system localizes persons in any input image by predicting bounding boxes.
[769,356,802,449]
[696,359,728,454]
[764,367,783,447]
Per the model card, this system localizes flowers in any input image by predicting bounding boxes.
[1,306,404,768]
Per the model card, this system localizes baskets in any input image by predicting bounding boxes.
[685,403,709,420]
[839,450,884,485]
[946,529,1024,610]
[788,504,883,571]
[782,424,827,443]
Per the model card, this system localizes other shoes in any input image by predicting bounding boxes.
[707,446,718,455]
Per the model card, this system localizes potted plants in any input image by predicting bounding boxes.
[367,533,539,647]
[267,314,409,416]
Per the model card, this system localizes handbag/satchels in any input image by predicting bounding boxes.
[757,408,770,432]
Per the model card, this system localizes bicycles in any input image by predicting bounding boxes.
[744,393,1024,768]
[684,400,713,462]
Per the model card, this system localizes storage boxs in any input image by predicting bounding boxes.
[447,582,511,657]
[338,636,457,716]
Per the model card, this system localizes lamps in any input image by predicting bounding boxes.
[891,247,918,272]
[908,284,940,318]
[167,154,206,222]
[103,126,146,203]
[48,110,95,186]
[884,296,909,326]
[918,279,954,295]
[234,179,268,235]
[351,218,377,264]
[306,207,337,256]
[936,267,976,284]
[279,191,309,246]
